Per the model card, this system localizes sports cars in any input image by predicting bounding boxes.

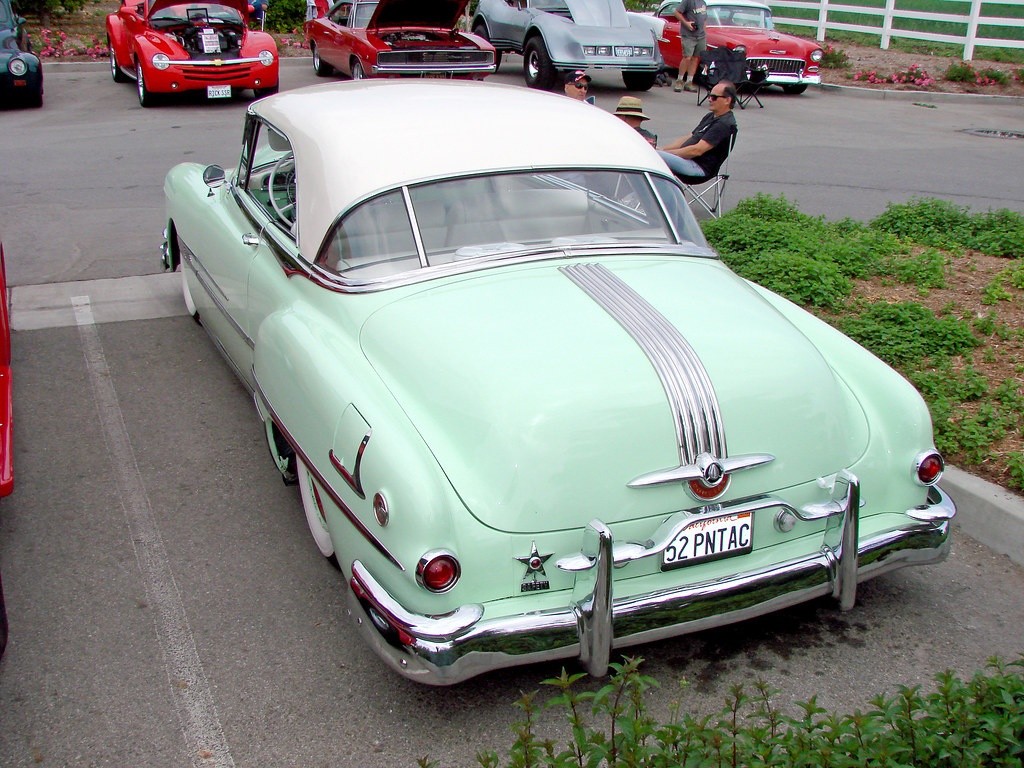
[303,0,498,80]
[105,0,281,108]
[470,0,666,91]
[627,0,825,94]
[0,0,44,109]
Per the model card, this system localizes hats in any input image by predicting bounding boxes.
[613,95,649,120]
[564,71,591,83]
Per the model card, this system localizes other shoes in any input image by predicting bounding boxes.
[656,78,663,87]
[663,72,672,86]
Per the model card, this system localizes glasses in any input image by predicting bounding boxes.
[568,83,588,90]
[708,94,727,101]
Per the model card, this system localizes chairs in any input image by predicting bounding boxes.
[326,198,448,269]
[697,66,774,109]
[613,124,738,218]
[449,189,589,246]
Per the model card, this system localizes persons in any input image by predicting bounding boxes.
[674,0,707,92]
[580,96,657,197]
[305,0,335,23]
[655,79,737,185]
[564,70,592,104]
[247,0,270,21]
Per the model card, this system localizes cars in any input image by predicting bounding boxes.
[157,77,958,689]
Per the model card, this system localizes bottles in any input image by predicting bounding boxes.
[710,62,715,75]
[758,66,761,71]
[702,65,708,75]
[746,69,751,80]
[763,64,768,79]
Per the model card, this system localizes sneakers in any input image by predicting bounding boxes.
[675,79,683,91]
[684,81,697,92]
